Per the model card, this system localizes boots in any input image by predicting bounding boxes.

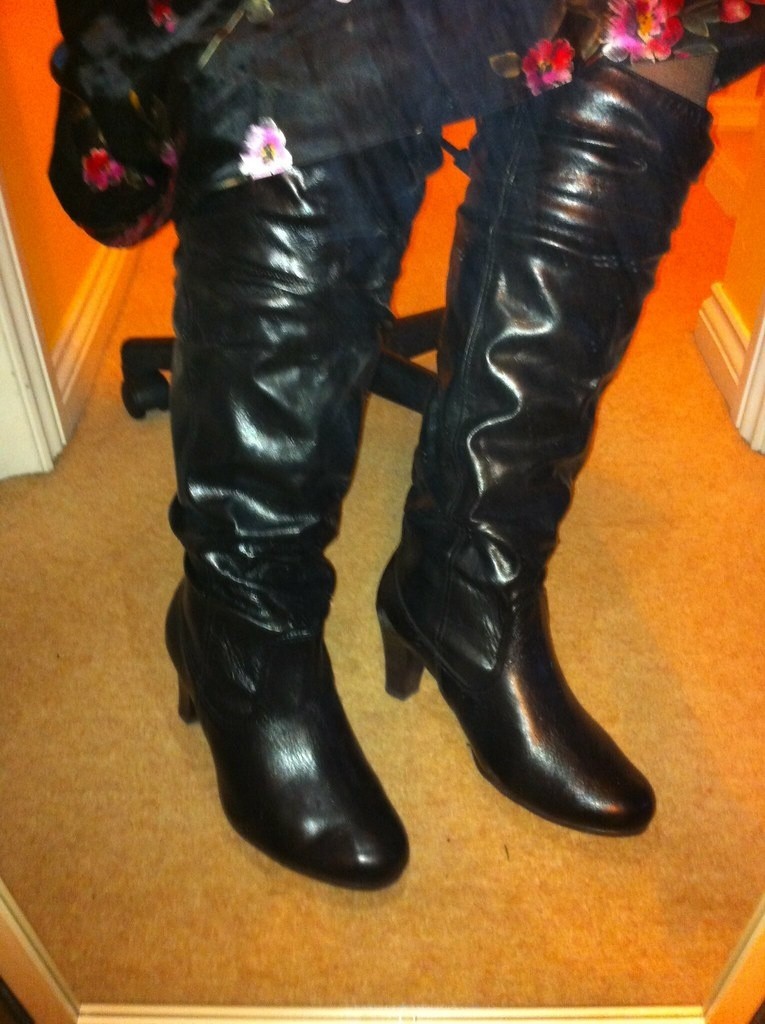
[373,57,714,837]
[165,118,441,893]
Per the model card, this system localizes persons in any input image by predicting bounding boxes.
[48,0,765,890]
